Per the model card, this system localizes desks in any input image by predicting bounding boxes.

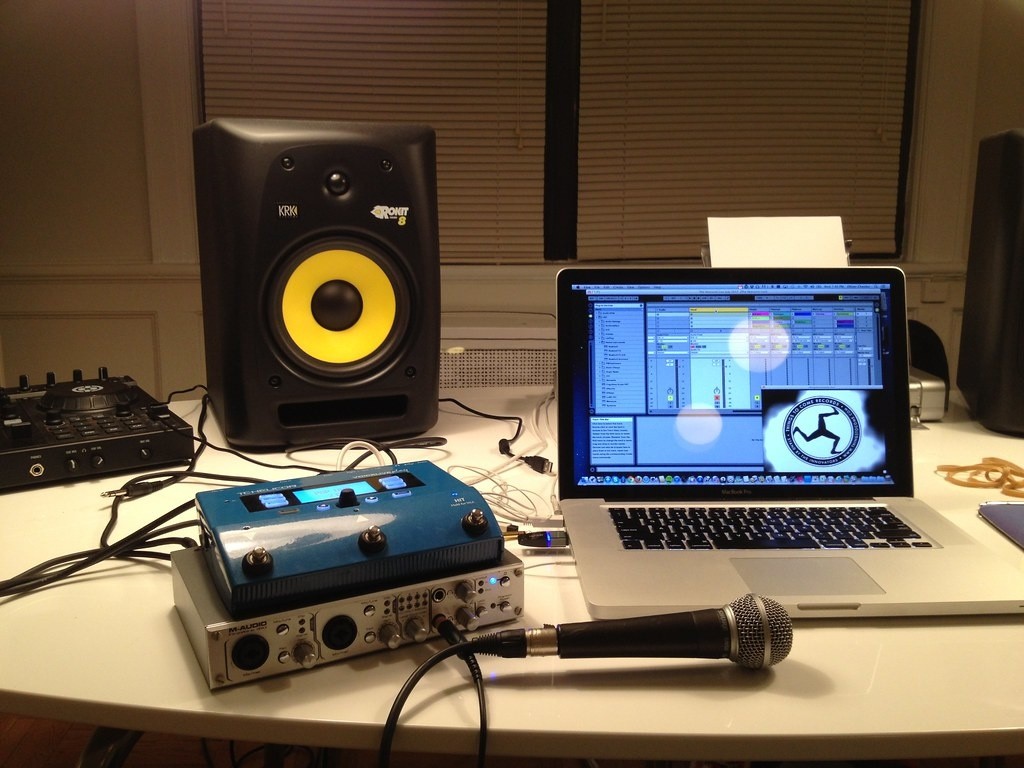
[1,387,1024,768]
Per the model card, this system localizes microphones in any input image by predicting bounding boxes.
[475,594,794,668]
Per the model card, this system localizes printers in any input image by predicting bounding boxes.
[698,240,944,432]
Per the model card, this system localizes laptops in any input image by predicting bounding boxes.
[550,267,1024,620]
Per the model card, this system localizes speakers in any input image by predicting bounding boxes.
[957,130,1024,437]
[191,119,442,447]
